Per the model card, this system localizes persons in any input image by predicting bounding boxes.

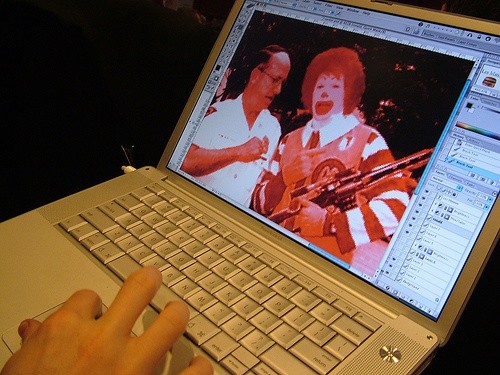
[0,265,218,375]
[250,46,410,256]
[179,45,291,210]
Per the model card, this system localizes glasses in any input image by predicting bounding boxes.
[258,67,287,86]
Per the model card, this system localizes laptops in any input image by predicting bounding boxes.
[0,0,500,375]
